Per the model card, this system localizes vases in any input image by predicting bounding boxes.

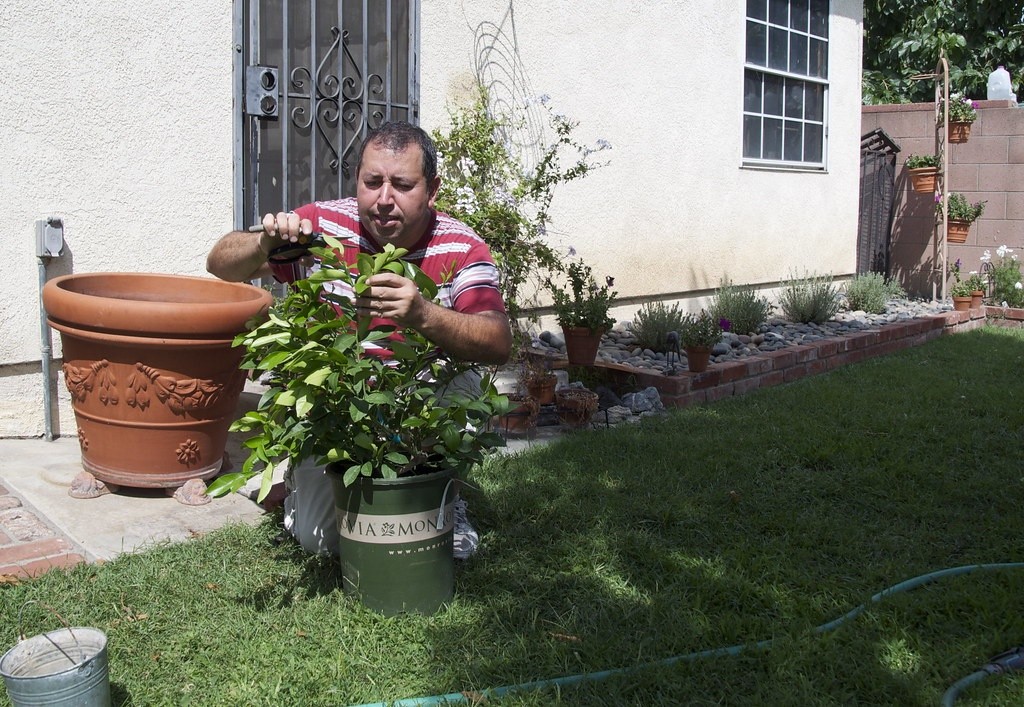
[686,346,712,372]
[43,272,272,505]
[940,217,970,243]
[948,121,974,143]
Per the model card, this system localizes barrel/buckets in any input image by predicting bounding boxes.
[0,600,112,707]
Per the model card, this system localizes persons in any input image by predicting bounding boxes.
[207,121,513,561]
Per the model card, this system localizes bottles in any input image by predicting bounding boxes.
[987,66,1012,101]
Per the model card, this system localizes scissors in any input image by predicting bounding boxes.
[267,235,360,263]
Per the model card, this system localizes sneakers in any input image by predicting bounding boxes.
[283,490,300,542]
[450,498,478,559]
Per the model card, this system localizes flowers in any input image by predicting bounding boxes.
[934,193,988,227]
[937,92,980,128]
[679,309,732,350]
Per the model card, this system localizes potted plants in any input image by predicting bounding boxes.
[905,153,942,193]
[951,274,989,311]
[486,257,618,440]
[202,232,522,618]
